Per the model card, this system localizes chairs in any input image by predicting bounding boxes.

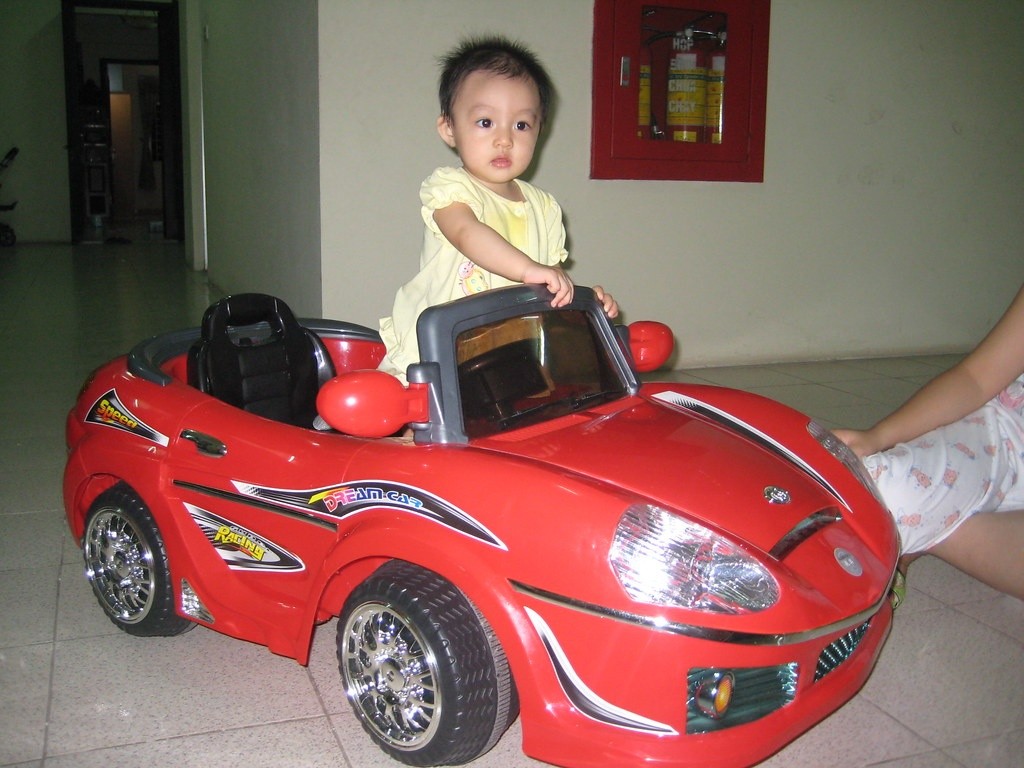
[187,292,336,433]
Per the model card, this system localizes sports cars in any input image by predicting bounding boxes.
[60,277,904,767]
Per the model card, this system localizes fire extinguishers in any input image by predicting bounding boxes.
[635,14,728,144]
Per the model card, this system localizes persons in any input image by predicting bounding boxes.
[376,35,620,441]
[831,288,1024,607]
